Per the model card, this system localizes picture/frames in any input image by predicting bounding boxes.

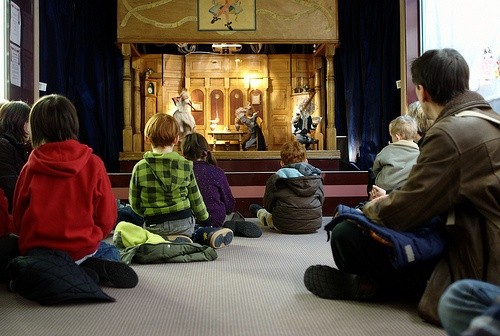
[197,0,257,31]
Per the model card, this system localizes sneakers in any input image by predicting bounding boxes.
[224,220,262,238]
[81,258,138,288]
[158,234,194,246]
[249,204,264,218]
[303,265,377,303]
[419,262,451,321]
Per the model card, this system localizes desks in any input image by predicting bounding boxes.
[208,132,247,151]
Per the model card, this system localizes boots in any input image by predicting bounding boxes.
[196,226,233,249]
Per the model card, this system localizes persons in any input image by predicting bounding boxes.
[302,48,500,329]
[0,88,500,336]
